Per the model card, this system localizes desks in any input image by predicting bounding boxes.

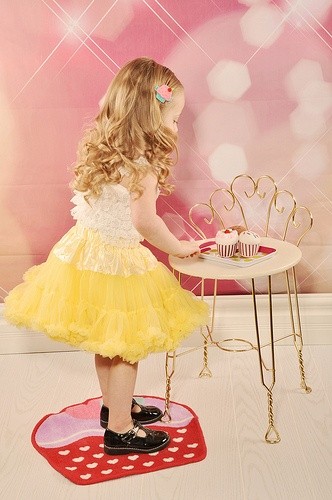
[164,174,316,444]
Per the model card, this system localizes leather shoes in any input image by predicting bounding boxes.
[104,419,169,455]
[100,398,164,428]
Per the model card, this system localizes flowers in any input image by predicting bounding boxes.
[154,85,176,103]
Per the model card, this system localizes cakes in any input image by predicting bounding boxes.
[214,225,261,257]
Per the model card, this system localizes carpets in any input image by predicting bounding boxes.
[31,395,207,485]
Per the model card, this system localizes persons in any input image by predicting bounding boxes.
[0,56,213,457]
